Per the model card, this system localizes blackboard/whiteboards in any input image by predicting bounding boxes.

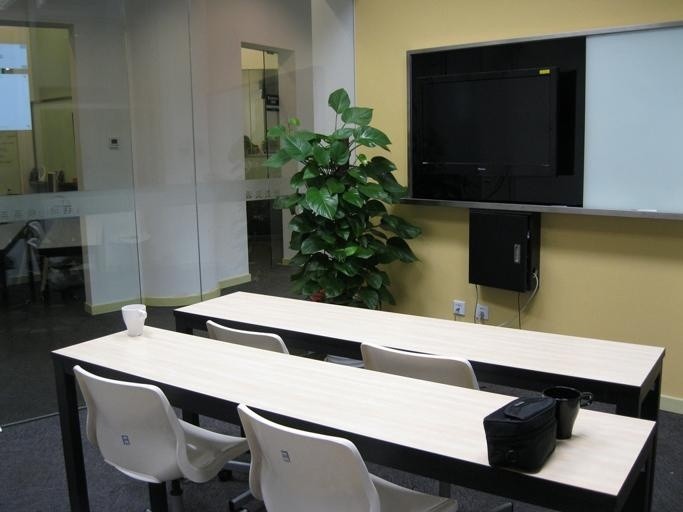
[583,20,683,220]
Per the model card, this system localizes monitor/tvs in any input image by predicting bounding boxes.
[415,67,558,178]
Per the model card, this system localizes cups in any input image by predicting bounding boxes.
[540,386,593,439]
[121,304,147,337]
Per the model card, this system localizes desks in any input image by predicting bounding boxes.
[52,326,658,511]
[174,291,666,419]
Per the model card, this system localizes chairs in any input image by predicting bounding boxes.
[205,321,327,511]
[71,363,249,511]
[359,343,514,511]
[237,404,462,510]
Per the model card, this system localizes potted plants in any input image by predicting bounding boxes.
[261,88,421,362]
